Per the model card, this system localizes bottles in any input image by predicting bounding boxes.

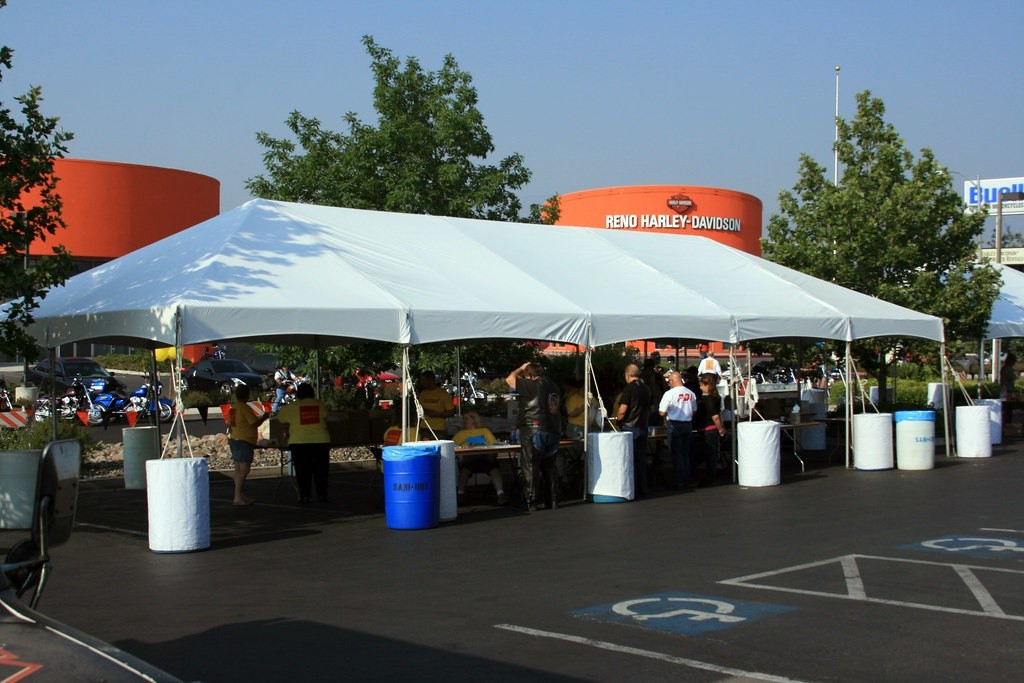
[510,426,517,443]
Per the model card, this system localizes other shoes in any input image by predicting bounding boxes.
[698,481,714,488]
[298,497,309,505]
[512,501,537,513]
[233,496,254,505]
[547,498,558,507]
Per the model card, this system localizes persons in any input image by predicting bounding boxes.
[269,364,297,418]
[616,363,651,498]
[592,351,765,422]
[277,383,330,504]
[1000,353,1018,421]
[199,343,226,359]
[659,371,697,489]
[229,384,269,504]
[417,371,455,440]
[698,344,709,359]
[800,361,824,389]
[696,373,726,486]
[451,412,512,505]
[512,363,561,510]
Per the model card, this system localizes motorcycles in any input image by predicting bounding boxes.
[1,376,176,426]
[439,366,486,406]
[266,376,310,407]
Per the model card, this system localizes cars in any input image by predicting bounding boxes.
[245,353,285,376]
[336,369,405,390]
[29,357,130,401]
[746,360,869,385]
[175,358,265,401]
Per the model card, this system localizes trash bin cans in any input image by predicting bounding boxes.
[956,406,992,458]
[401,440,458,521]
[587,431,634,502]
[869,387,892,405]
[801,389,826,449]
[145,458,210,554]
[927,383,950,408]
[122,426,160,488]
[895,411,935,470]
[737,420,781,486]
[970,399,1001,444]
[853,414,893,470]
[382,446,444,529]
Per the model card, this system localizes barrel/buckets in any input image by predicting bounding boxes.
[737,420,781,487]
[852,383,1002,471]
[402,440,458,522]
[587,431,634,503]
[146,456,211,554]
[0,450,39,529]
[121,426,158,490]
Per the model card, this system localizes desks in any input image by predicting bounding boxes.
[380,421,820,523]
[263,441,384,502]
[814,417,851,464]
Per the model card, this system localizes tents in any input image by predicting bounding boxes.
[940,261,1024,379]
[0,197,950,457]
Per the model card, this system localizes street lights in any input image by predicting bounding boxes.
[833,65,840,261]
[10,209,31,277]
